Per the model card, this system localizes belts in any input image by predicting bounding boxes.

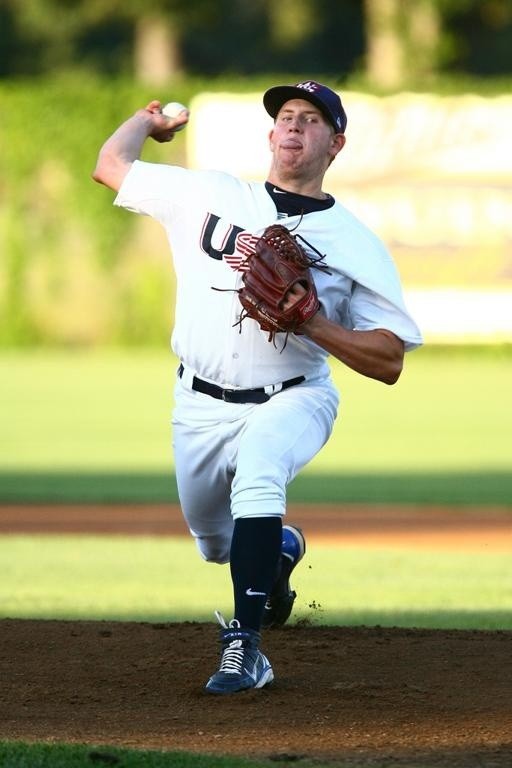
[180,364,305,403]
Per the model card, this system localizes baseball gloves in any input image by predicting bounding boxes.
[209,207,331,356]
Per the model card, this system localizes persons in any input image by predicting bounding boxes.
[87,75,424,700]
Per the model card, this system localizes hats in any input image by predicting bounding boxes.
[264,80,346,134]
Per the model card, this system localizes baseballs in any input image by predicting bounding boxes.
[163,103,188,130]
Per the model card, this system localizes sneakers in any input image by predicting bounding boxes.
[206,611,274,694]
[261,525,305,626]
